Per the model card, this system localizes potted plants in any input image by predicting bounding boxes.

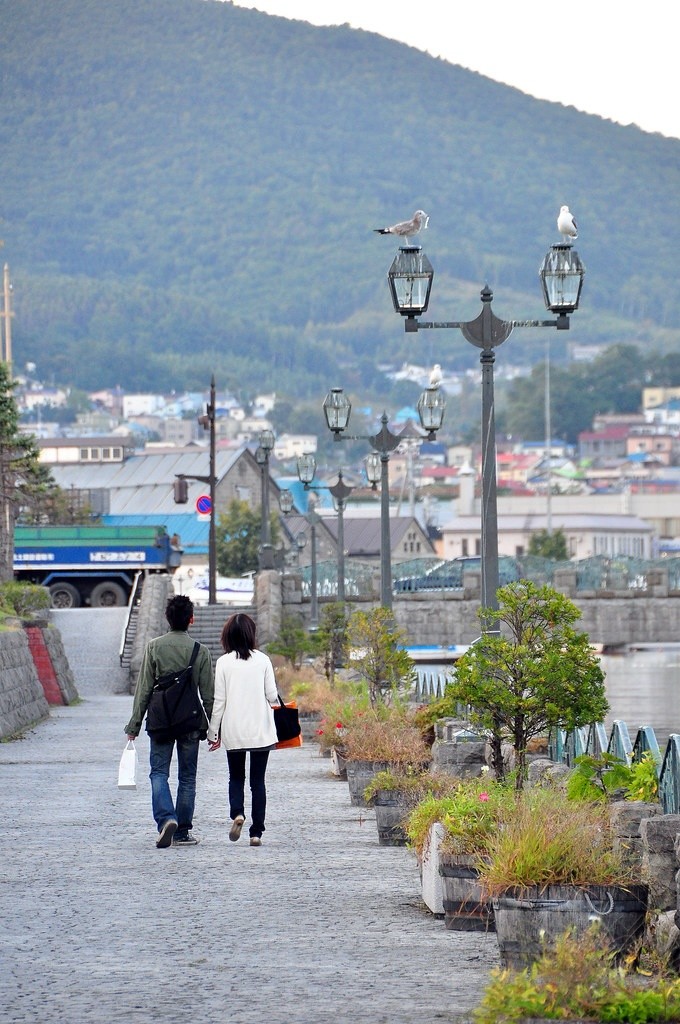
[293,684,341,744]
[346,722,432,805]
[362,768,461,847]
[475,794,680,1024]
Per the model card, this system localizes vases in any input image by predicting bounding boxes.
[421,821,521,919]
[332,744,347,778]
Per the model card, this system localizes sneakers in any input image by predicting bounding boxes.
[173,833,200,846]
[156,817,178,848]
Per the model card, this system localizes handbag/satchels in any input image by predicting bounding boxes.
[144,640,204,732]
[117,739,139,791]
[269,695,301,741]
[268,698,302,749]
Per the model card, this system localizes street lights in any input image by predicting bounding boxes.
[297,452,380,671]
[254,431,275,569]
[389,239,585,642]
[279,488,348,637]
[322,378,447,649]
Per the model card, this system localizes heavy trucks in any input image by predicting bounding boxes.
[10,523,184,610]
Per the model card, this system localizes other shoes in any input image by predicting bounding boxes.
[249,837,263,846]
[229,814,245,842]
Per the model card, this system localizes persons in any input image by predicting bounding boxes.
[123,594,215,847]
[207,612,278,846]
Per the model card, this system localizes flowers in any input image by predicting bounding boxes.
[406,766,538,865]
[316,706,382,747]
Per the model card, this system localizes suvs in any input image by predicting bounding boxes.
[391,553,528,591]
